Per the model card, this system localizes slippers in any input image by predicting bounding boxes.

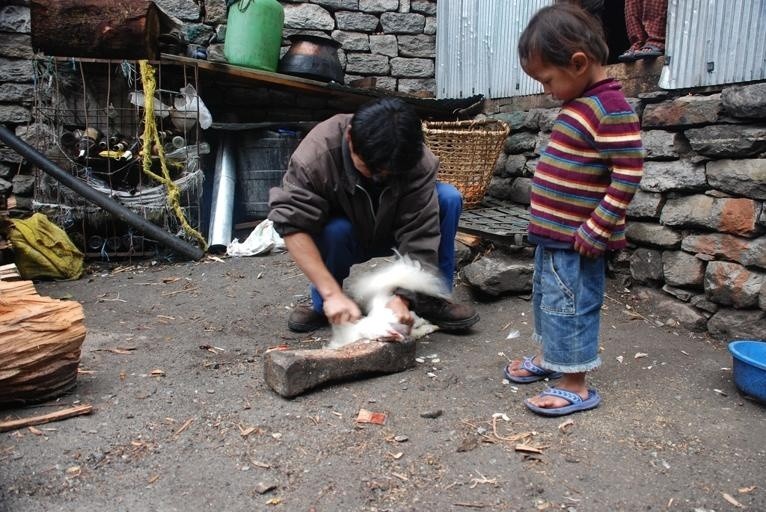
[502,356,559,383]
[524,384,602,417]
[619,49,640,63]
[636,43,664,58]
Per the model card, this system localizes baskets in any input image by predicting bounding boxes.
[422,115,509,210]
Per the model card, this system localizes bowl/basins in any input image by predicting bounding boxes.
[275,34,345,85]
[729,340,766,404]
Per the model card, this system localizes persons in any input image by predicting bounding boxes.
[619,1,668,63]
[268,97,480,333]
[504,6,642,417]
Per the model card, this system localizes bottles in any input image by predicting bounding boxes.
[60,126,186,165]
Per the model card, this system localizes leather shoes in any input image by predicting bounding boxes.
[287,294,328,331]
[414,296,481,330]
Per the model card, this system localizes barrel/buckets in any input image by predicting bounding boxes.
[236,137,306,218]
[223,0,284,73]
